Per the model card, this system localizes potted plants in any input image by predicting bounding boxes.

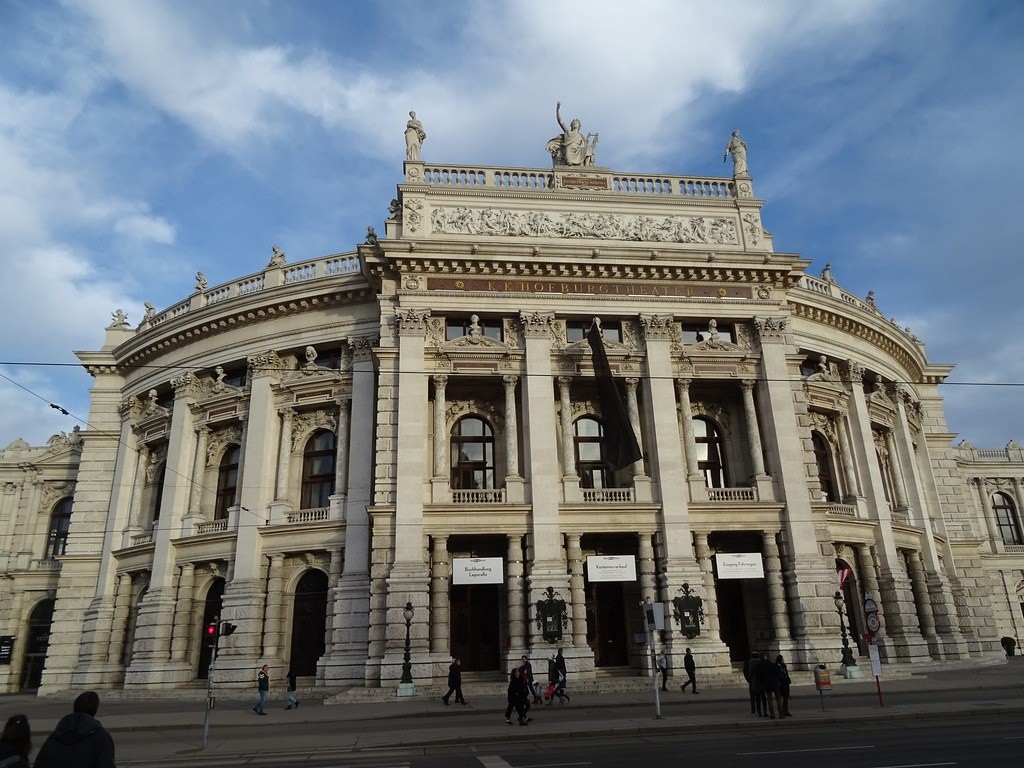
[1001,637,1016,656]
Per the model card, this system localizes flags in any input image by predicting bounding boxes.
[587,321,644,473]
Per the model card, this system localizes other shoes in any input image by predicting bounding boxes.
[455,700,461,703]
[505,721,514,726]
[784,712,792,717]
[545,701,554,706]
[443,696,449,705]
[770,716,775,719]
[294,700,300,709]
[780,715,786,718]
[562,698,565,704]
[258,712,268,715]
[662,687,668,692]
[526,718,533,722]
[763,713,769,717]
[252,707,258,713]
[519,721,528,726]
[532,701,538,704]
[462,702,468,705]
[692,690,700,694]
[284,705,293,710]
[681,684,685,693]
[567,696,570,702]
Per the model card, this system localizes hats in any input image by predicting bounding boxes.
[547,652,555,660]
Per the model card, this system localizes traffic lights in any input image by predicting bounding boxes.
[207,622,217,645]
[219,622,232,636]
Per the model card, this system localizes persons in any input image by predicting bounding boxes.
[144,302,155,317]
[742,648,793,719]
[906,397,914,409]
[215,366,227,381]
[284,666,299,710]
[0,714,32,768]
[872,374,885,391]
[149,389,158,400]
[556,101,598,166]
[252,665,270,715]
[592,316,602,331]
[817,356,828,370]
[387,199,402,219]
[32,692,117,768]
[822,263,835,280]
[365,225,377,244]
[470,315,479,324]
[112,310,128,328]
[195,272,207,286]
[546,648,571,706]
[504,655,544,726]
[404,110,425,161]
[680,648,700,694]
[305,345,317,358]
[708,318,718,333]
[441,658,468,705]
[272,246,285,260]
[656,648,669,692]
[725,130,749,177]
[865,291,876,309]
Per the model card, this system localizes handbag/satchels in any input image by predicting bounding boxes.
[558,670,565,682]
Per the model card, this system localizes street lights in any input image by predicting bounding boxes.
[399,600,415,683]
[833,590,857,666]
